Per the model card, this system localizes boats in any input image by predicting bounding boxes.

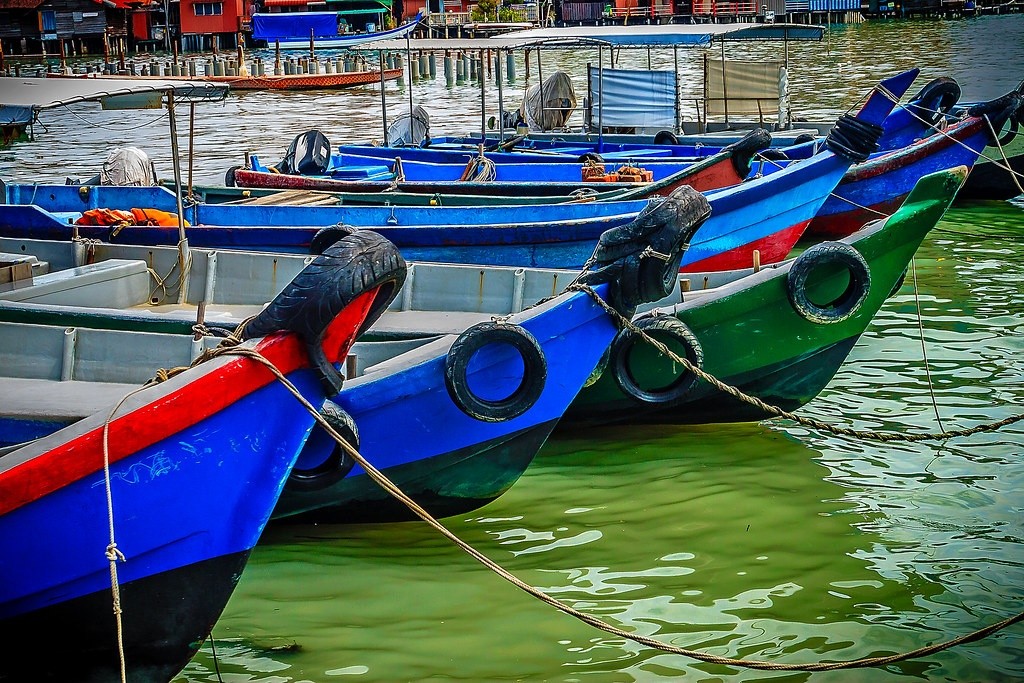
[0,229,405,683]
[1,0,1024,284]
[1,182,711,526]
[1,171,976,433]
